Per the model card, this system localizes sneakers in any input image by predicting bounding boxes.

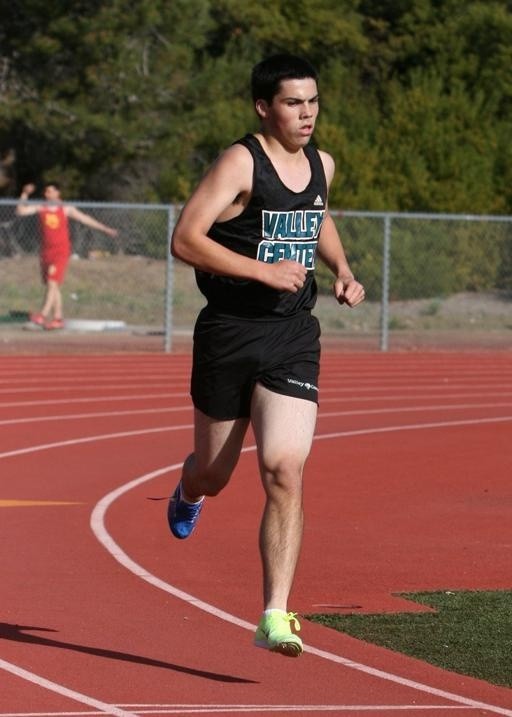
[168,479,206,539]
[255,610,303,657]
[28,312,65,329]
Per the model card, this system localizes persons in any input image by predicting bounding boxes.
[0,146,24,256]
[16,183,118,330]
[169,55,367,655]
